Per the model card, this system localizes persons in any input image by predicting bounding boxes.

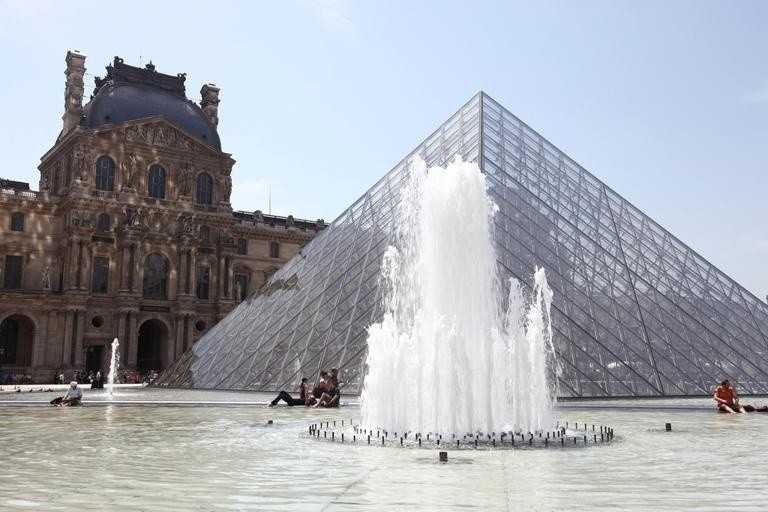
[266,366,348,410]
[53,381,83,408]
[1,368,161,390]
[714,379,749,413]
